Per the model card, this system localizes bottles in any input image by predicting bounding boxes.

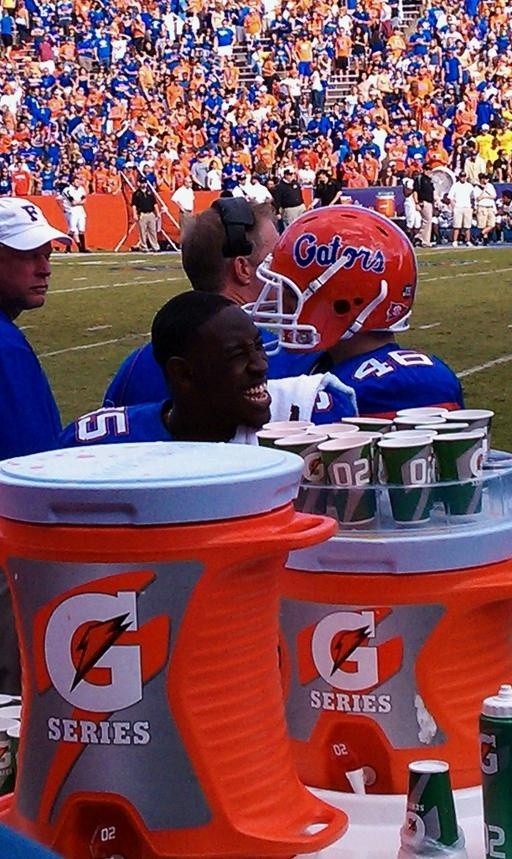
[478,686,511,859]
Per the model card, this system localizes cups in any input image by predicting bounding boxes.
[255,408,494,528]
[399,759,462,851]
[0,693,24,799]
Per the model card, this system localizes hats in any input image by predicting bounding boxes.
[0,0,512,184]
[0,196,74,254]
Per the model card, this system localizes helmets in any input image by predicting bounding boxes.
[266,203,419,352]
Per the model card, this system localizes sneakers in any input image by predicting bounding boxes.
[412,231,504,248]
[139,247,162,253]
[63,249,94,253]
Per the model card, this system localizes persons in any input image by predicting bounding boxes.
[0,0,512,252]
[54,290,273,450]
[241,201,467,426]
[99,196,326,407]
[1,197,77,461]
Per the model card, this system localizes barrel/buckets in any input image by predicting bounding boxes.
[280,490,512,796]
[1,439,349,859]
[374,191,396,219]
